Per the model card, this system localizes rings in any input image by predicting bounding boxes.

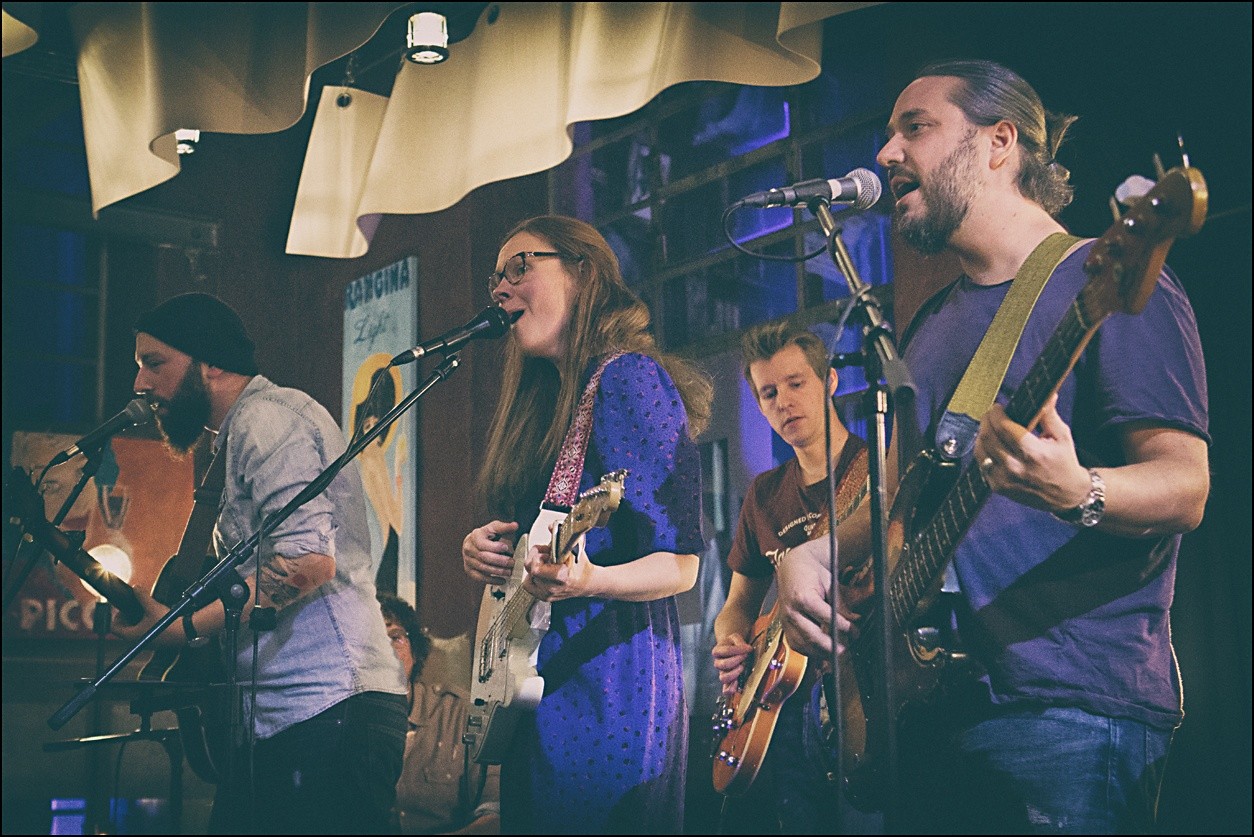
[980,459,994,474]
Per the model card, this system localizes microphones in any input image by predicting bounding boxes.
[50,399,154,466]
[392,307,512,365]
[740,168,883,209]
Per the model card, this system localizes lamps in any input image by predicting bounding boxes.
[175,128,201,155]
[404,2,449,64]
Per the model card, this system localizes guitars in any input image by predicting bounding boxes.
[131,546,244,783]
[708,165,1210,799]
[463,463,634,770]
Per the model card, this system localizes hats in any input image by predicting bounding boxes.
[133,292,259,376]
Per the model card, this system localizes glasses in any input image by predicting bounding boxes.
[487,251,560,299]
[387,628,412,648]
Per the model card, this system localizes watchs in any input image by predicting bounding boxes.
[1051,467,1106,529]
[182,613,211,651]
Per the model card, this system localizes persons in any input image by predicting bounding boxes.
[713,319,876,837]
[373,592,502,837]
[109,290,410,837]
[462,215,710,837]
[777,60,1211,837]
